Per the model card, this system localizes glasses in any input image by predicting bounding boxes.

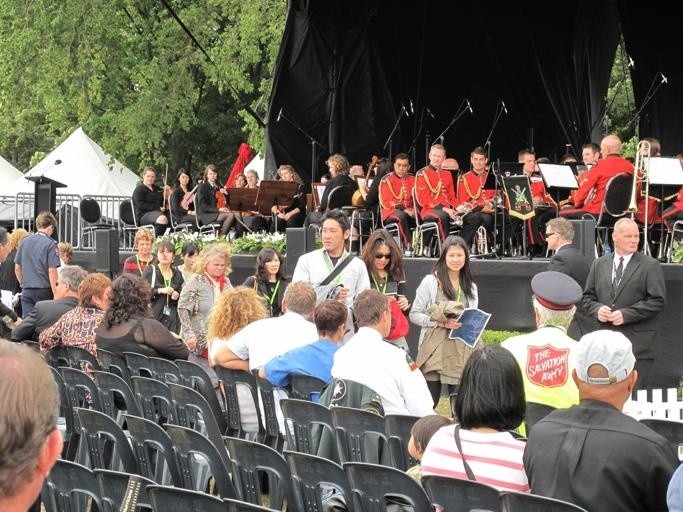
[542,232,554,238]
[374,255,391,259]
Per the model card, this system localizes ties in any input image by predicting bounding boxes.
[610,257,623,296]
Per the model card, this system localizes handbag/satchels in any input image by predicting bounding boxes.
[385,299,409,340]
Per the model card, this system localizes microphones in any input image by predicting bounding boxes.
[502,101,508,114]
[400,103,409,117]
[426,108,435,118]
[276,106,283,122]
[629,56,636,70]
[660,72,671,86]
[410,100,414,113]
[466,99,473,113]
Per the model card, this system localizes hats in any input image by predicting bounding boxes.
[574,329,634,385]
[531,270,583,311]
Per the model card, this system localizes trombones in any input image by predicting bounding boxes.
[623,140,650,256]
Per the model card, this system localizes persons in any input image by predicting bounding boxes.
[1,210,287,368]
[579,216,669,402]
[0,336,64,511]
[521,327,680,511]
[542,216,592,342]
[501,270,584,410]
[358,228,411,313]
[258,299,348,403]
[132,131,680,260]
[331,287,435,418]
[387,416,453,511]
[290,207,370,342]
[418,341,531,511]
[407,234,480,421]
[213,279,322,379]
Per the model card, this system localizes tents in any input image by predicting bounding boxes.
[2,130,144,221]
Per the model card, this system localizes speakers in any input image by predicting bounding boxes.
[569,219,595,261]
[286,227,312,277]
[96,229,119,281]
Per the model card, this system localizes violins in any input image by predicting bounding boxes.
[160,173,172,212]
[214,191,226,208]
[180,177,202,210]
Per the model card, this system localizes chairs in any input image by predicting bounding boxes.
[23,344,683,512]
[81,158,683,260]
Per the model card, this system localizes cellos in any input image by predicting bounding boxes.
[350,155,383,228]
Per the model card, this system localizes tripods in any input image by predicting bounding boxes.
[469,176,504,260]
[504,219,551,261]
